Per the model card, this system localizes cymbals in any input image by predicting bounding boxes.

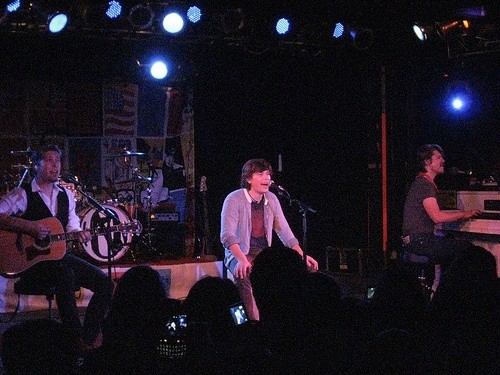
[101,151,143,157]
[11,149,35,156]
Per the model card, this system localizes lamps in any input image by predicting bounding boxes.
[348,22,373,48]
[413,19,465,43]
[30,0,71,35]
[127,5,154,30]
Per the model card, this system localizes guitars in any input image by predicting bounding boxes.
[198,175,216,255]
[0,216,143,279]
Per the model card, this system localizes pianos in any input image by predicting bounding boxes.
[403,172,500,278]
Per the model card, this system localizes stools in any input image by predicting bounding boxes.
[10,275,80,321]
[402,253,436,302]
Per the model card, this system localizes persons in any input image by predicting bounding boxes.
[219,158,319,325]
[0,144,114,356]
[401,143,498,306]
[0,244,500,375]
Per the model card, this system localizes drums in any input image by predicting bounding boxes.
[77,204,136,263]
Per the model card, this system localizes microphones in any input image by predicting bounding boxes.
[449,167,465,175]
[62,172,81,186]
[269,180,283,195]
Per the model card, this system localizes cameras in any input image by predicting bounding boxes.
[226,301,249,326]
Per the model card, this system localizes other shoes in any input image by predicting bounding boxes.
[76,338,97,356]
